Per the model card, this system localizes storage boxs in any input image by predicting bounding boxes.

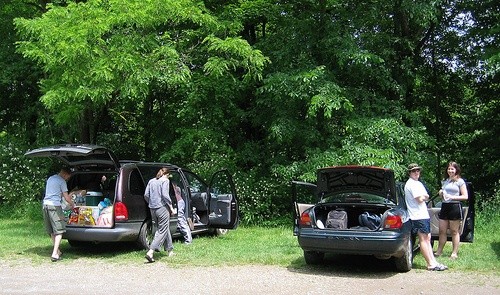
[78,206,99,226]
[85,192,103,206]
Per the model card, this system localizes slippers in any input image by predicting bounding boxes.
[145,255,155,262]
[429,264,448,271]
[52,258,63,262]
[59,252,63,256]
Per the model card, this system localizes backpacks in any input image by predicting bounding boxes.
[359,212,381,230]
[326,211,347,230]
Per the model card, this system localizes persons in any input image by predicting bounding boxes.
[143,168,176,263]
[169,179,192,244]
[434,162,468,260]
[42,167,75,262]
[404,163,448,271]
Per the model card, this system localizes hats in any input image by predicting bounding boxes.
[407,164,422,170]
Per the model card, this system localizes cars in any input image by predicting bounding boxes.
[23,142,241,252]
[290,163,476,272]
